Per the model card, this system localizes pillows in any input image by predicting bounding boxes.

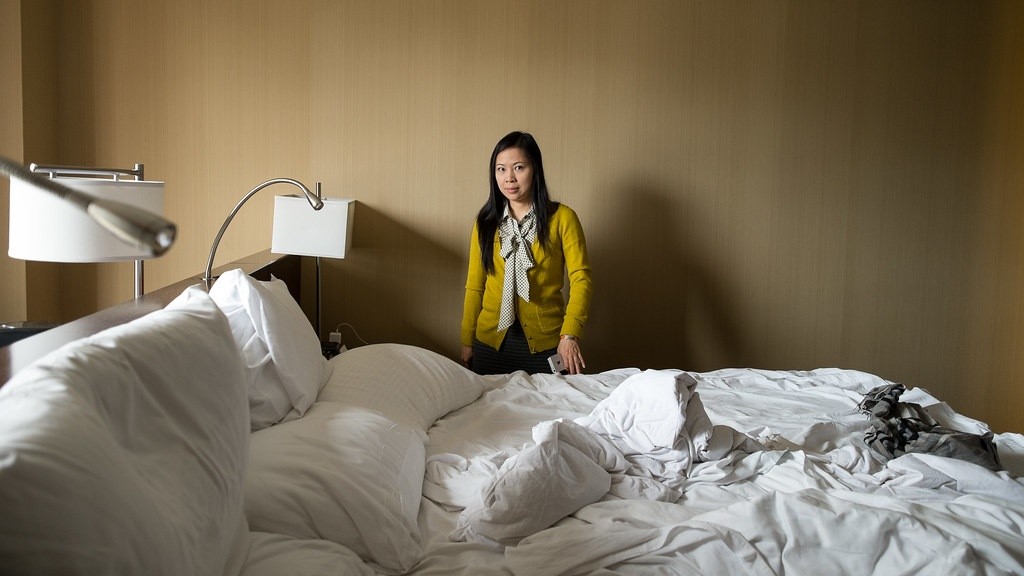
[318,343,490,433]
[244,403,429,575]
[1,284,250,576]
[205,268,334,432]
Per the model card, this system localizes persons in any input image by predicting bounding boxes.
[460,131,593,374]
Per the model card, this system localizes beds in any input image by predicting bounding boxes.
[1,247,1024,575]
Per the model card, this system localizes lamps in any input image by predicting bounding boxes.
[204,178,326,291]
[6,162,168,302]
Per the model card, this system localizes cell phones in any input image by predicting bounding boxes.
[547,353,566,374]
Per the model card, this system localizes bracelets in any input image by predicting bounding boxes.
[560,334,580,341]
